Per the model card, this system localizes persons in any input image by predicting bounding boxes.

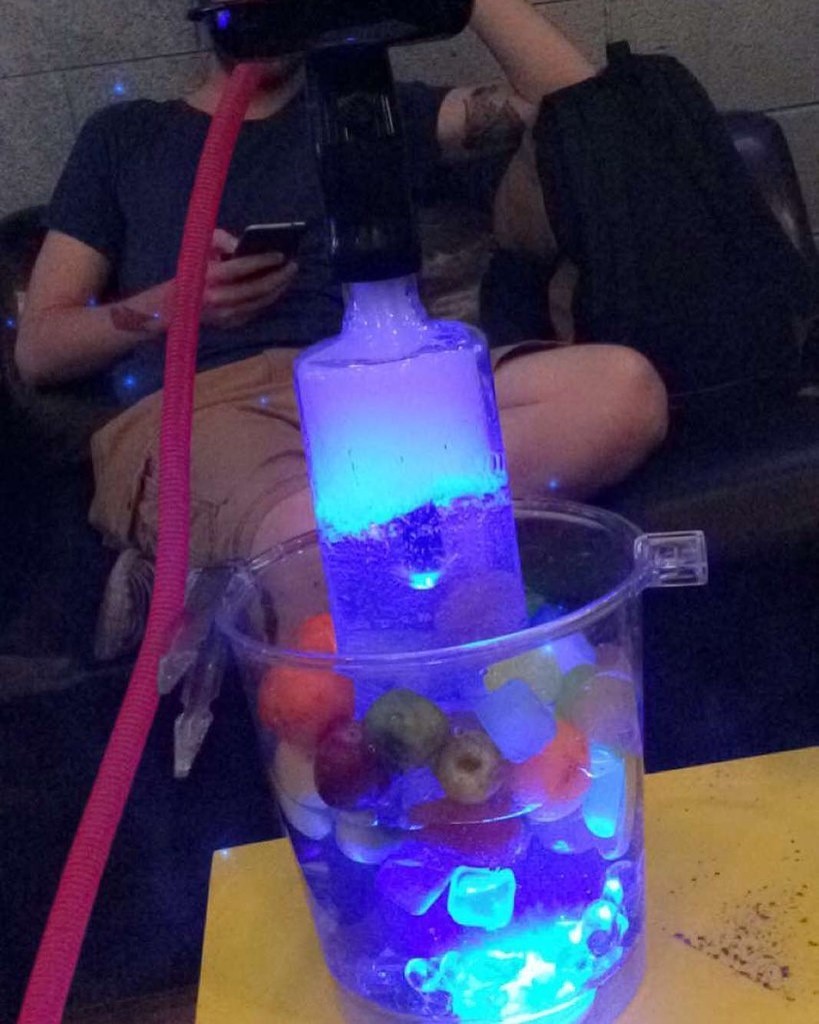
[13,0,671,840]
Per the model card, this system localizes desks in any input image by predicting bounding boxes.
[196,747,819,1024]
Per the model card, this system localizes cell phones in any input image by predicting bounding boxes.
[229,221,305,260]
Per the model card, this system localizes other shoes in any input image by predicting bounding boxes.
[95,548,156,661]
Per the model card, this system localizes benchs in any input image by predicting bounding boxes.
[1,112,818,703]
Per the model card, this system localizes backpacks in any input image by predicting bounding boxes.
[534,39,819,401]
